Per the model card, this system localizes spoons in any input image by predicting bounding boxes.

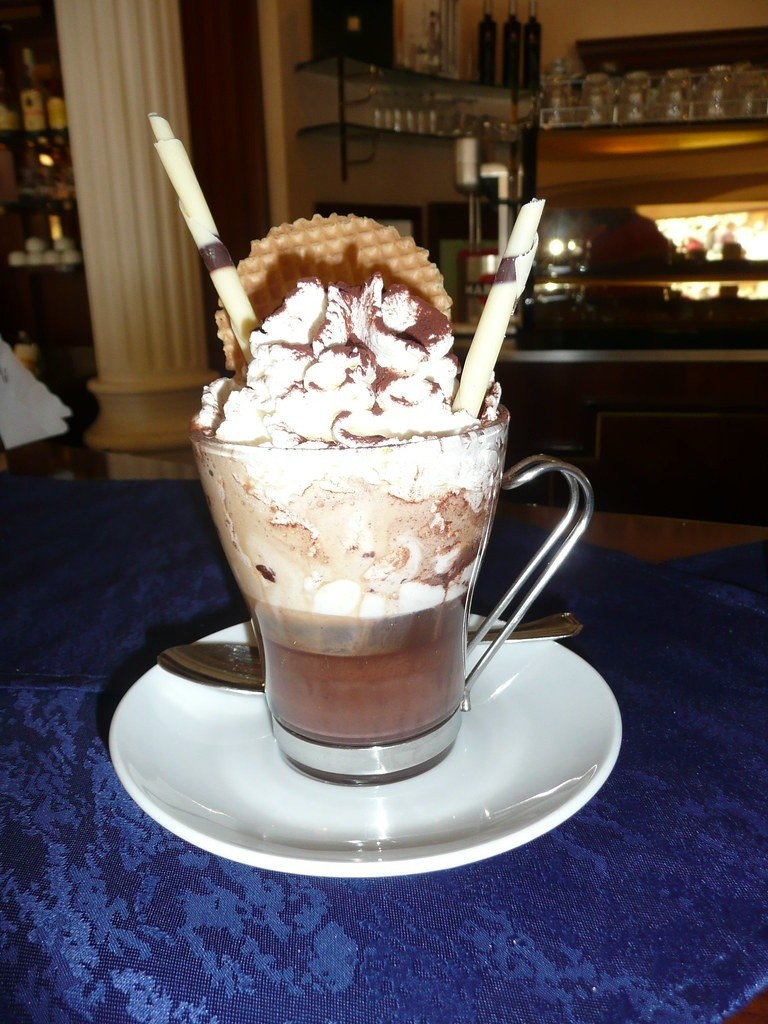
[161,612,586,690]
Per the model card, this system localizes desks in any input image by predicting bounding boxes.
[0,481,768,1024]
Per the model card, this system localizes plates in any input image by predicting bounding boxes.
[109,614,624,882]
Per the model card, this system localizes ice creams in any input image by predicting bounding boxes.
[187,213,510,618]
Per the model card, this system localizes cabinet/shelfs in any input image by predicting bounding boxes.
[293,49,768,363]
[0,0,92,350]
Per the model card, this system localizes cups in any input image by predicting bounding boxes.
[190,407,594,785]
[545,65,768,124]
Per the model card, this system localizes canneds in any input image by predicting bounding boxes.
[46,96,66,129]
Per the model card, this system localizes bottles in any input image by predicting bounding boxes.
[43,47,67,131]
[523,0,542,89]
[479,0,496,86]
[503,0,522,89]
[19,46,46,130]
